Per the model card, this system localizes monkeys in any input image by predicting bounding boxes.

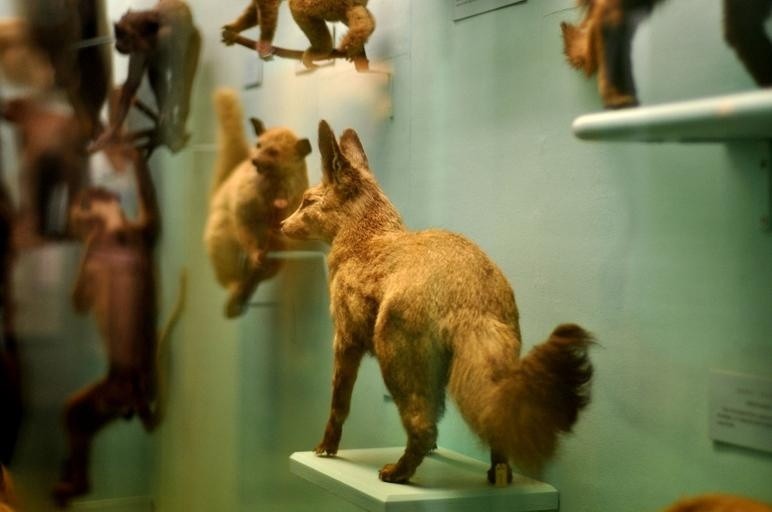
[84,0,204,155]
[45,143,188,501]
